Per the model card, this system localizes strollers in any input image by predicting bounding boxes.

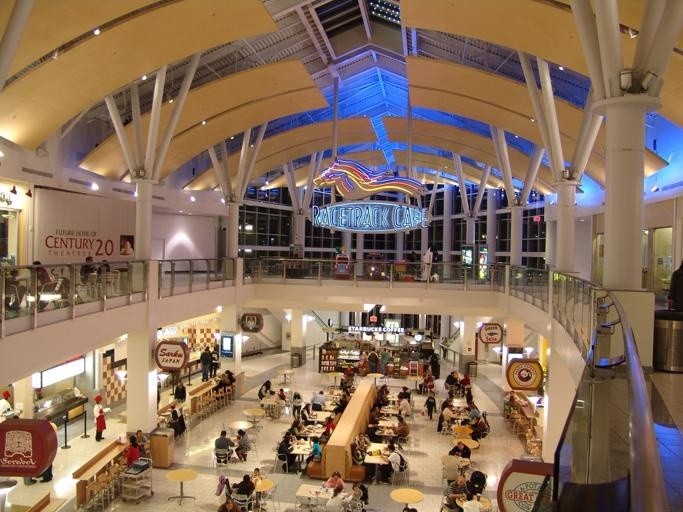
[1,270,25,315]
[50,267,83,308]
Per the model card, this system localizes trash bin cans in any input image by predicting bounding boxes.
[468,362,477,377]
[291,353,300,368]
[654,310,683,372]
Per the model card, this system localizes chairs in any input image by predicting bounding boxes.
[195,379,239,424]
[85,454,128,512]
[5,270,121,314]
[502,385,544,459]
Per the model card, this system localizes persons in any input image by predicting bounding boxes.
[440,474,484,512]
[120,239,134,256]
[430,244,439,275]
[164,402,185,429]
[506,396,517,419]
[278,365,355,472]
[92,395,109,444]
[0,389,14,418]
[173,379,187,403]
[37,413,59,485]
[200,346,235,386]
[136,429,151,449]
[215,429,251,464]
[442,372,486,459]
[219,468,262,512]
[124,434,143,469]
[325,472,362,512]
[666,256,683,314]
[352,384,411,481]
[29,259,53,310]
[409,251,418,272]
[368,347,417,374]
[258,380,271,407]
[503,390,519,402]
[424,392,436,419]
[0,257,26,312]
[79,256,99,286]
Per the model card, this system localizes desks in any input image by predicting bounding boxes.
[215,371,491,512]
[150,426,175,470]
[166,468,198,505]
[73,430,127,508]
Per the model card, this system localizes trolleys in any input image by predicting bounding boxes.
[118,457,154,507]
